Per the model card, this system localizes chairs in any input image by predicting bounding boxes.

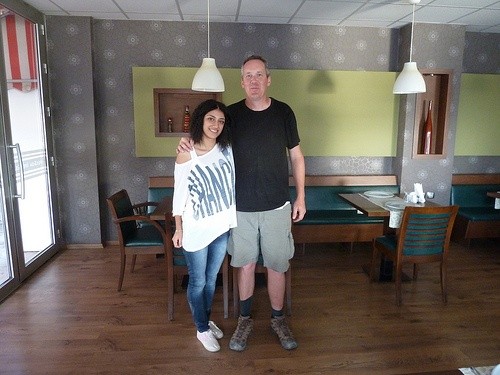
[164,211,229,320]
[109,189,182,291]
[234,253,292,319]
[377,206,458,305]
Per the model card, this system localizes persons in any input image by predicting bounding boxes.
[172,98,237,352]
[176,55,306,351]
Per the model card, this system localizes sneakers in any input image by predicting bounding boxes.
[229,315,255,351]
[196,329,220,352]
[207,321,223,339]
[270,315,297,349]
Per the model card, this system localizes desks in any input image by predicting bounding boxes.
[338,193,441,282]
[150,197,174,220]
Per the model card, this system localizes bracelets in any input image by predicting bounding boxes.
[175,230,183,232]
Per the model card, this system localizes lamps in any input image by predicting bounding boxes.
[394,0,427,94]
[192,0,224,92]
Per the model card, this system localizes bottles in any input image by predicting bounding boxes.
[184,105,191,133]
[168,117,173,133]
[421,101,433,154]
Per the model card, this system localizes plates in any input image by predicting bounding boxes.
[363,191,395,199]
[386,201,409,210]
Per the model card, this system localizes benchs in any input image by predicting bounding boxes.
[452,174,500,247]
[147,175,399,257]
